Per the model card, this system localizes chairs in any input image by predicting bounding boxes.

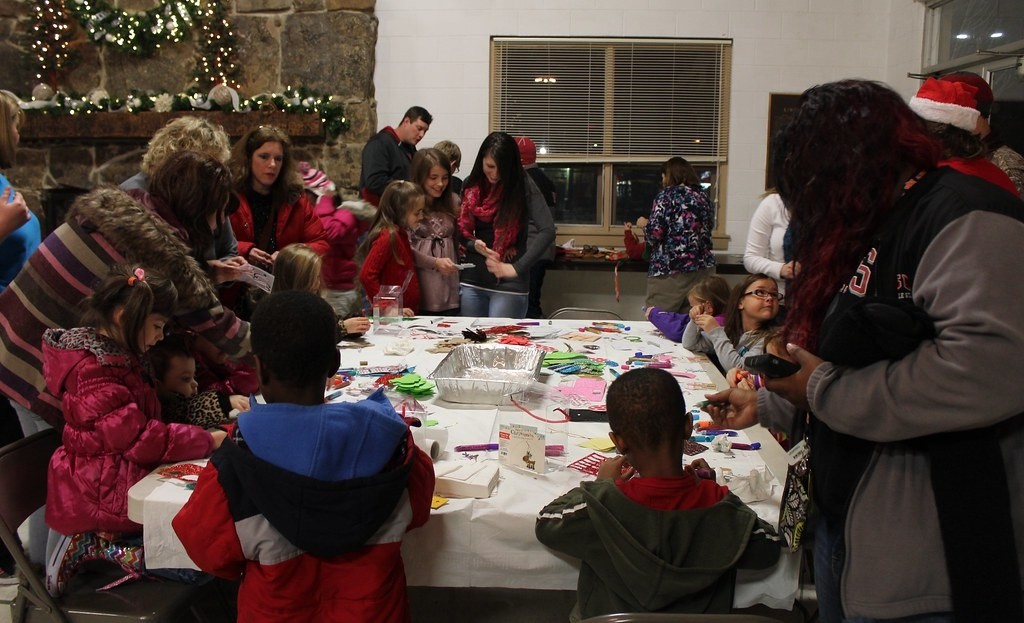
[0,428,231,623]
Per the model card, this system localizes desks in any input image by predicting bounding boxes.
[127,315,817,623]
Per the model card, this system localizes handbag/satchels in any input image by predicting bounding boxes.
[778,454,809,548]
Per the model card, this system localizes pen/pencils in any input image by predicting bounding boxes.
[610,368,621,377]
[516,322,539,326]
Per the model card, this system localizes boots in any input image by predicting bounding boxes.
[46,533,144,598]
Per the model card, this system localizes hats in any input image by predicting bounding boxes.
[514,138,537,165]
[299,161,336,204]
[908,77,981,137]
[942,71,994,110]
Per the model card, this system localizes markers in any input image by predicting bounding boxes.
[693,400,723,408]
[455,444,499,451]
[325,390,343,403]
[629,355,673,367]
[585,322,625,335]
[545,445,564,456]
[731,442,755,450]
[362,309,366,317]
[701,430,738,437]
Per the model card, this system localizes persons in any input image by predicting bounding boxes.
[0,106,555,602]
[682,73,1024,623]
[0,91,43,587]
[637,156,717,315]
[171,290,436,623]
[535,368,782,623]
[645,275,731,343]
[623,222,649,269]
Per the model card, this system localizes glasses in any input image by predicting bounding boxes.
[744,289,783,301]
[454,167,460,173]
[581,245,599,255]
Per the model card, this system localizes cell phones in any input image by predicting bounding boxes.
[745,353,801,378]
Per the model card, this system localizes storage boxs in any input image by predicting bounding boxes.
[428,344,546,407]
